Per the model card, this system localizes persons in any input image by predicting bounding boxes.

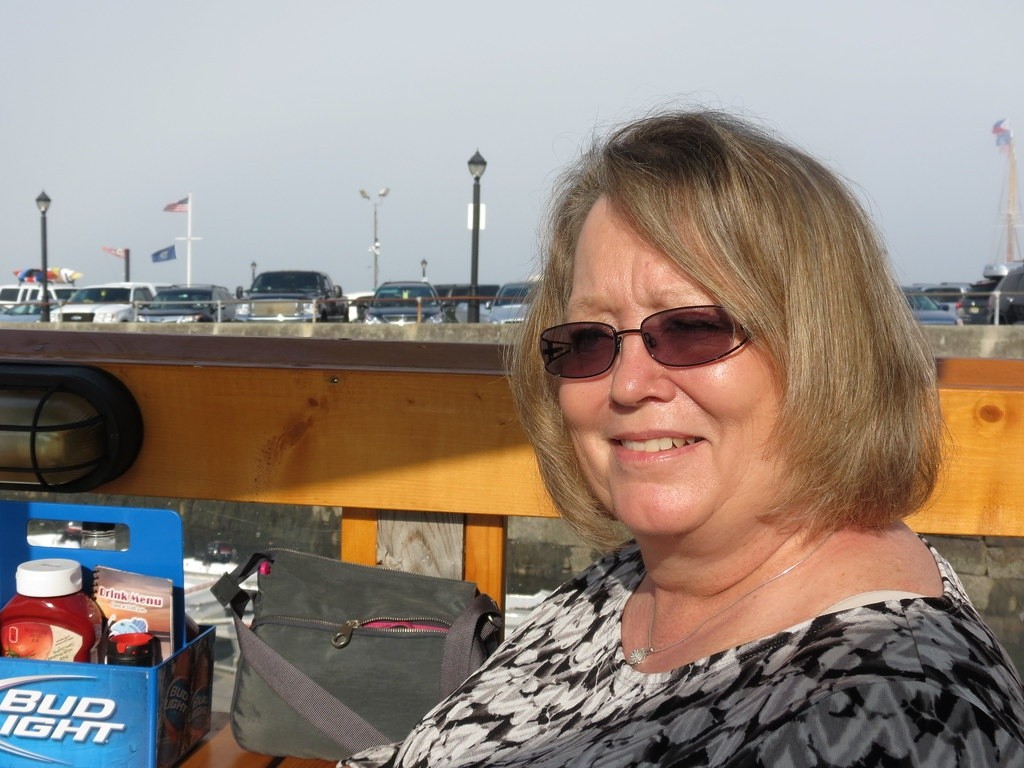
[334,109,1024,768]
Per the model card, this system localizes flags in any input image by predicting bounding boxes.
[164,195,188,212]
[152,245,176,263]
[103,246,125,259]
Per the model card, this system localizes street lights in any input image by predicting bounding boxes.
[359,187,391,296]
[34,189,52,323]
[466,150,489,324]
[249,261,258,282]
[421,257,427,280]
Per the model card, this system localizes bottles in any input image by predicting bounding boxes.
[0,558,102,665]
[106,633,157,667]
[80,522,116,550]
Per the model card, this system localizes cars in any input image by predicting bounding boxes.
[898,288,963,326]
[913,281,974,314]
[139,285,236,323]
[955,262,1024,326]
[233,270,350,325]
[455,279,543,328]
[47,281,183,323]
[433,283,458,300]
[442,283,497,321]
[364,281,453,324]
[200,540,236,562]
[346,295,374,324]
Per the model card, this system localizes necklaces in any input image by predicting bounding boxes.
[629,531,834,668]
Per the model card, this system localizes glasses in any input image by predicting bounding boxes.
[537,304,751,380]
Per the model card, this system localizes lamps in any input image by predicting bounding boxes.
[0,358,145,493]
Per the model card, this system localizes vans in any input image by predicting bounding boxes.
[0,283,83,316]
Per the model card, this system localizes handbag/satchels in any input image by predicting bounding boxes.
[211,548,503,764]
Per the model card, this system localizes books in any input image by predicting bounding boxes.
[92,565,174,664]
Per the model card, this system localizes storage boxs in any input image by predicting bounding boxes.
[0,498,218,768]
